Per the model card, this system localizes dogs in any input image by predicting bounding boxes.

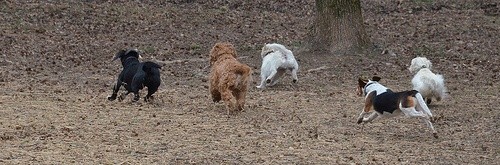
[355,75,439,139]
[107,48,163,104]
[408,56,446,105]
[208,42,254,115]
[255,42,299,89]
